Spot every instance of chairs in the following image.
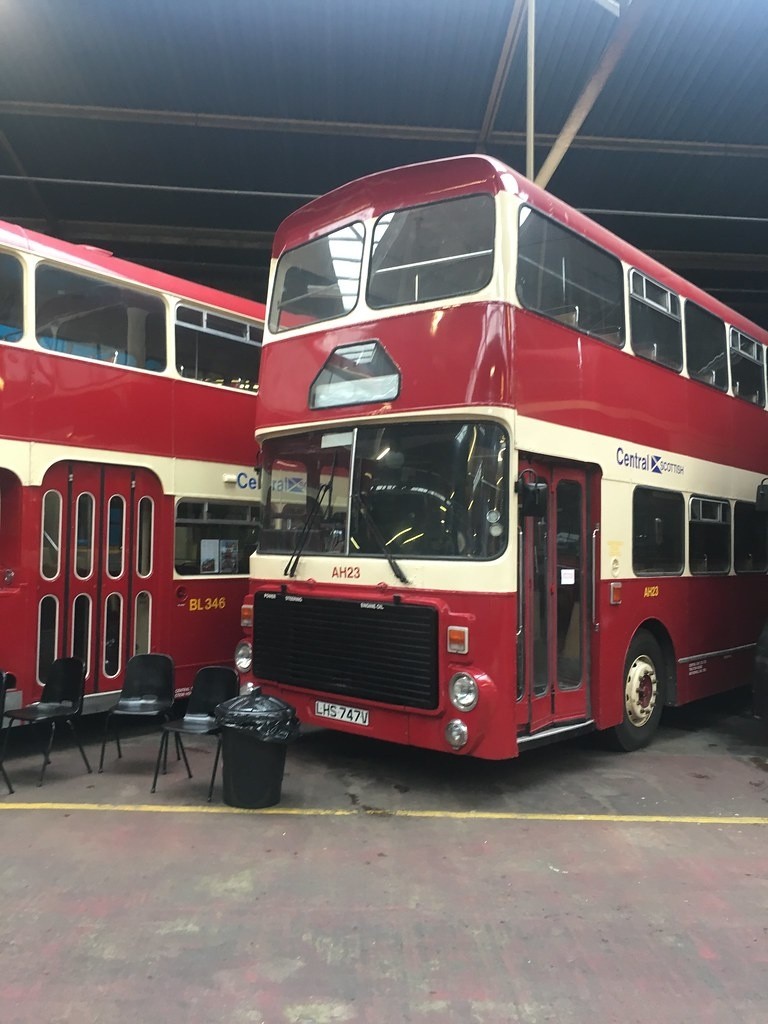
[0,657,93,794]
[151,665,240,801]
[97,653,180,774]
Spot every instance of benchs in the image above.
[540,306,760,405]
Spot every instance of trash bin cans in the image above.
[213,686,299,810]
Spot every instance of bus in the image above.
[0,220,344,730]
[236,152,768,762]
